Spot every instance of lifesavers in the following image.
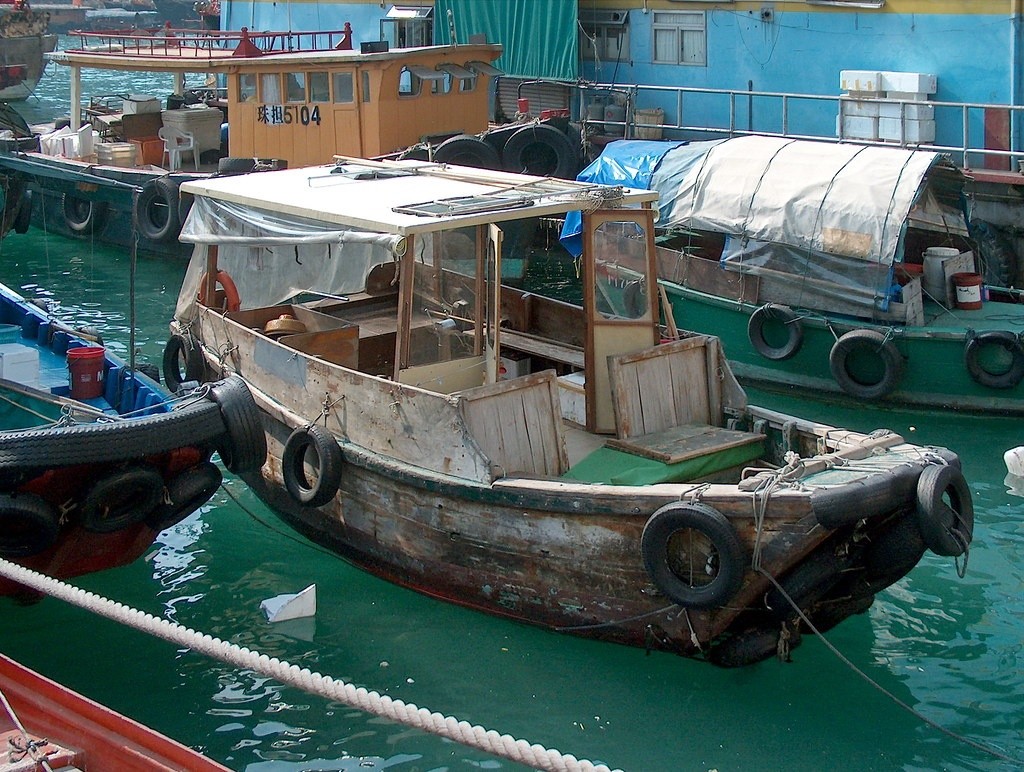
[828,329,903,401]
[16,190,33,234]
[30,299,49,313]
[0,327,268,557]
[937,215,1014,301]
[638,499,748,610]
[964,330,1024,390]
[281,423,344,508]
[746,302,804,361]
[61,191,107,236]
[218,157,288,173]
[431,123,580,181]
[131,178,180,244]
[762,464,975,620]
[200,269,241,313]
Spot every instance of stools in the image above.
[461,326,585,371]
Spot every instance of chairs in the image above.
[168,126,200,169]
[158,126,199,172]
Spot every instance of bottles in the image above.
[981,283,989,302]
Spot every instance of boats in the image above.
[162,151,977,671]
[2,0,1024,422]
[1,185,228,611]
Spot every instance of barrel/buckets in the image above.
[895,263,923,286]
[167,95,185,110]
[0,324,21,344]
[634,109,664,139]
[951,273,982,310]
[922,247,960,303]
[65,347,106,399]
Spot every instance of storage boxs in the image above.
[558,371,588,426]
[836,69,937,143]
[122,98,162,115]
[0,344,38,383]
[499,357,532,380]
[95,135,183,168]
